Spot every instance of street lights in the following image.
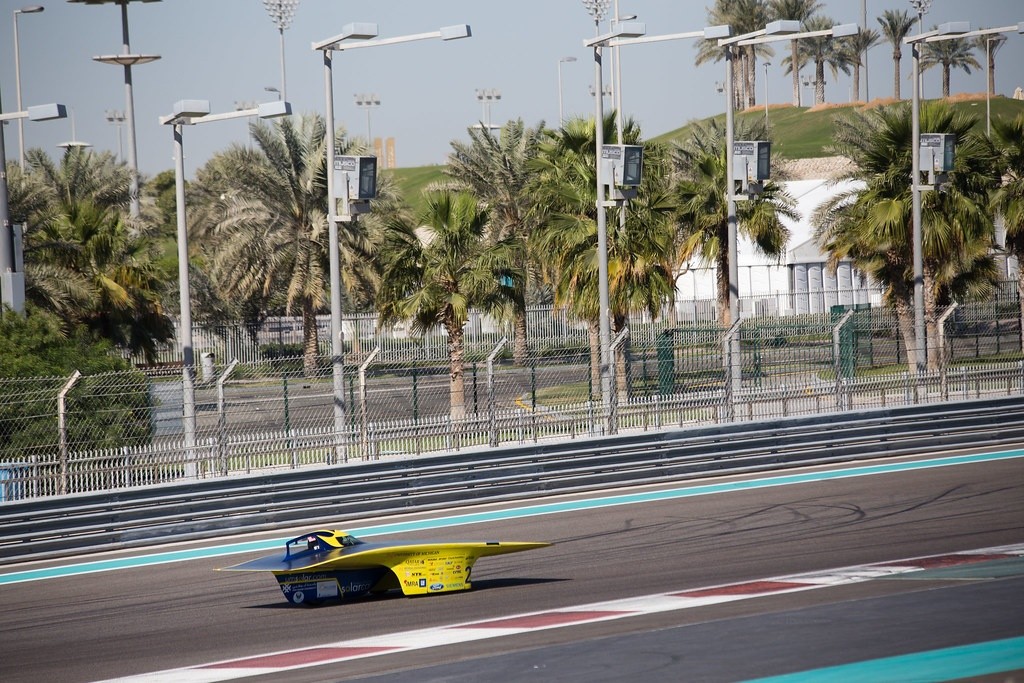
[909,0,932,99]
[264,86,282,101]
[763,62,772,141]
[0,93,67,323]
[902,20,1024,381]
[159,97,293,479]
[313,21,471,464]
[353,92,382,150]
[13,6,45,193]
[987,35,1008,139]
[560,56,578,129]
[106,109,128,164]
[611,14,639,120]
[584,19,733,442]
[716,19,862,419]
[475,87,502,137]
